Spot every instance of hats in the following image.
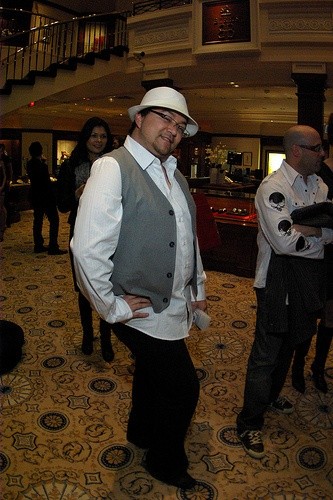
[127,87,199,140]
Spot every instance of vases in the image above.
[209,167,225,185]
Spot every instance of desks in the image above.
[9,180,57,211]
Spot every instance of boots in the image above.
[292,337,312,392]
[78,293,94,355]
[100,317,114,362]
[311,325,333,394]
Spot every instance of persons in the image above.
[70,87,208,489]
[56,117,114,362]
[0,144,13,242]
[292,114,333,394]
[236,126,333,458]
[26,141,67,255]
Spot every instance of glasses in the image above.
[149,109,190,139]
[297,144,328,152]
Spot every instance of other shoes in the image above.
[48,249,67,255]
[143,462,196,489]
[37,246,48,253]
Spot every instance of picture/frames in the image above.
[243,152,253,166]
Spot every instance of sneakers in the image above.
[238,429,265,459]
[268,397,294,414]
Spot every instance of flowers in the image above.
[204,143,228,169]
